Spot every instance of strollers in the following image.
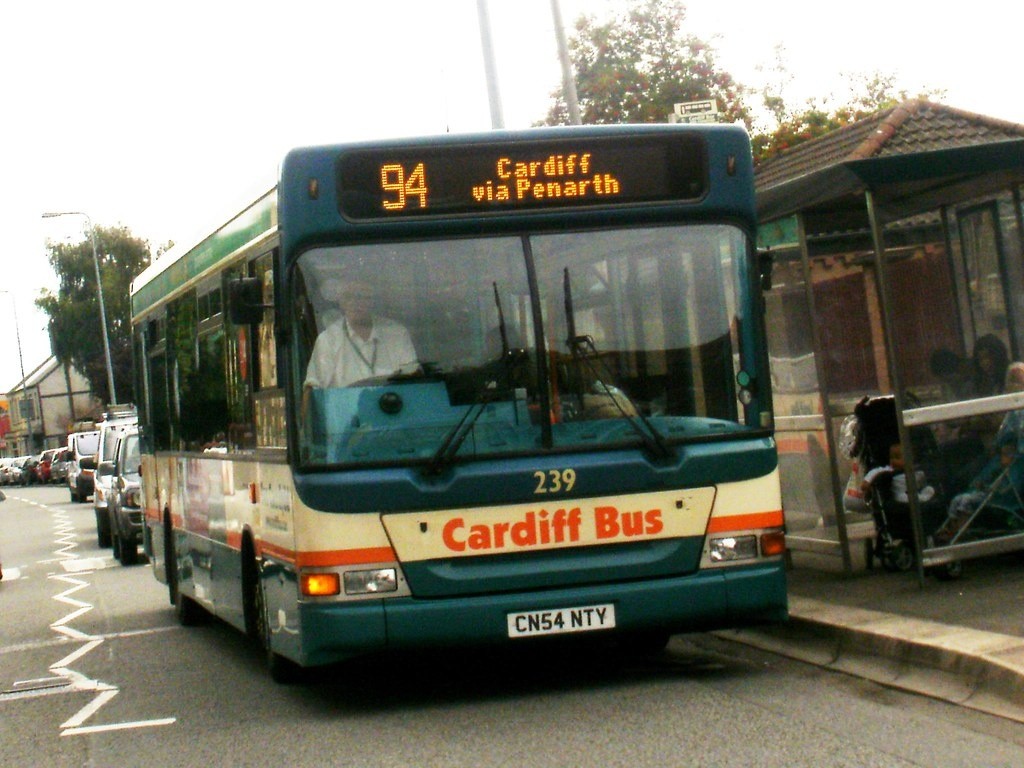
[849,390,949,572]
[924,427,1024,581]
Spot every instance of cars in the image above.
[0,445,71,488]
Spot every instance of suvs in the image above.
[98,431,143,567]
[92,416,140,549]
[65,429,103,504]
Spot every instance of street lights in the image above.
[39,210,117,405]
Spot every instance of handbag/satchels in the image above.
[843,456,867,514]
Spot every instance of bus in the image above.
[127,121,795,689]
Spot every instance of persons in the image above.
[860,334,1018,540]
[301,274,424,425]
